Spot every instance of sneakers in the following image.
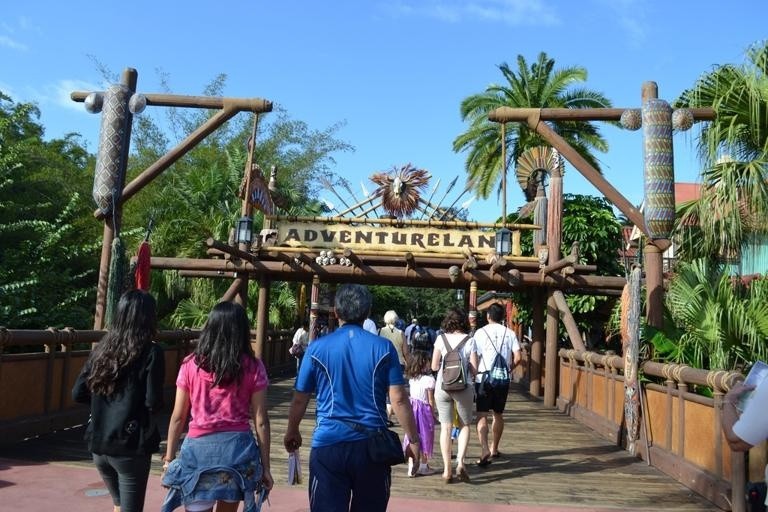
[409,464,436,478]
[475,451,511,469]
[441,468,472,485]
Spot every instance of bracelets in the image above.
[161,454,177,463]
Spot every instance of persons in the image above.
[430,307,479,485]
[402,349,439,478]
[287,301,462,458]
[720,374,767,512]
[71,288,166,512]
[283,282,421,512]
[161,300,275,512]
[472,302,523,467]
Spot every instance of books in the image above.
[732,359,768,413]
[285,443,303,486]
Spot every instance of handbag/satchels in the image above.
[410,326,416,341]
[369,427,405,465]
[291,344,304,358]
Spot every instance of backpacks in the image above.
[414,326,431,351]
[481,327,512,398]
[441,333,472,391]
